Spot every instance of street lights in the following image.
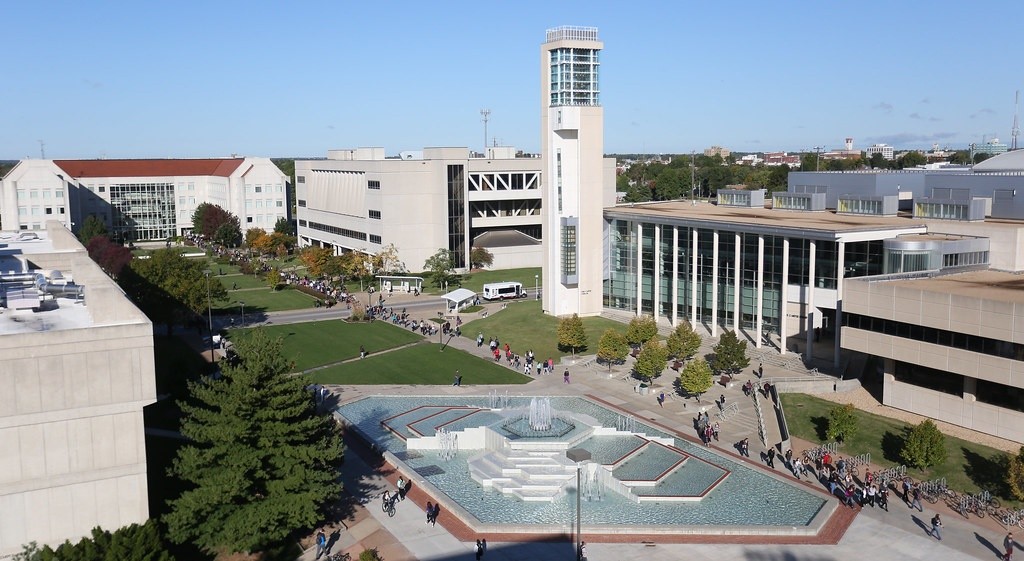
[202,269,216,379]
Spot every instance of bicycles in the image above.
[845,459,860,476]
[382,500,396,517]
[874,466,1024,531]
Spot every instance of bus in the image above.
[481,280,528,300]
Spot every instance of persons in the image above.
[475,294,480,305]
[397,476,405,502]
[190,234,347,301]
[459,284,462,288]
[453,369,462,387]
[382,490,392,510]
[504,343,521,368]
[548,357,554,370]
[1001,532,1014,561]
[476,332,483,349]
[414,288,418,296]
[474,538,486,561]
[442,321,461,337]
[483,312,489,318]
[489,336,499,351]
[563,368,570,384]
[659,363,944,541]
[360,345,365,359]
[543,359,549,375]
[580,541,587,561]
[316,529,329,559]
[426,500,435,528]
[492,346,500,362]
[537,361,542,375]
[524,349,534,375]
[366,293,436,336]
[502,303,506,308]
